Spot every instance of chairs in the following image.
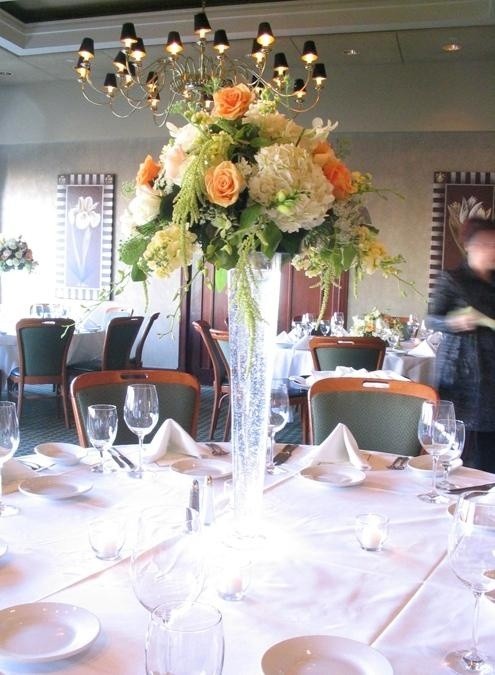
[68,365,200,452]
[191,315,439,456]
[0,302,160,433]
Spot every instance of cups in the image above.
[87,520,128,560]
[146,601,225,675]
[302,311,345,338]
[352,514,391,553]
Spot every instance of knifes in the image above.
[447,483,495,494]
[272,444,298,465]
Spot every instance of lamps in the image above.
[72,0,328,129]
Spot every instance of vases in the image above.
[221,251,284,509]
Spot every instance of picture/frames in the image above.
[427,168,494,322]
[56,172,114,302]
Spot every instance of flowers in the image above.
[0,231,39,272]
[59,73,430,361]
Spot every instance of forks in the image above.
[387,456,406,470]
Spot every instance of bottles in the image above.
[406,313,419,338]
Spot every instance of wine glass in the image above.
[445,491,495,674]
[131,509,207,675]
[268,384,289,466]
[0,400,22,518]
[86,384,159,474]
[386,335,401,353]
[418,399,465,504]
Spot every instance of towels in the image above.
[136,418,214,463]
[308,422,368,469]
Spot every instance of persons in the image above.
[424,218,495,474]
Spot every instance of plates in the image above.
[0,601,101,665]
[173,457,232,481]
[408,455,464,476]
[260,635,395,675]
[19,442,93,501]
[446,503,495,532]
[298,465,368,488]
